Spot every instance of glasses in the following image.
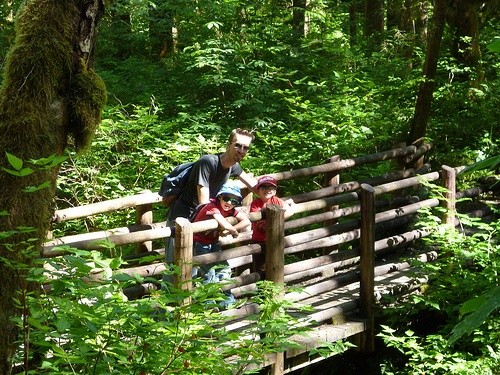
[222,195,239,207]
[258,185,274,191]
[231,141,249,150]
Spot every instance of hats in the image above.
[255,175,277,190]
[216,181,243,199]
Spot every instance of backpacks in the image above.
[158,154,217,208]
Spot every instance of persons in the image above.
[164,128,294,307]
[193,183,251,307]
[249,176,294,281]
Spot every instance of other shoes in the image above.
[209,306,220,315]
[234,297,248,309]
